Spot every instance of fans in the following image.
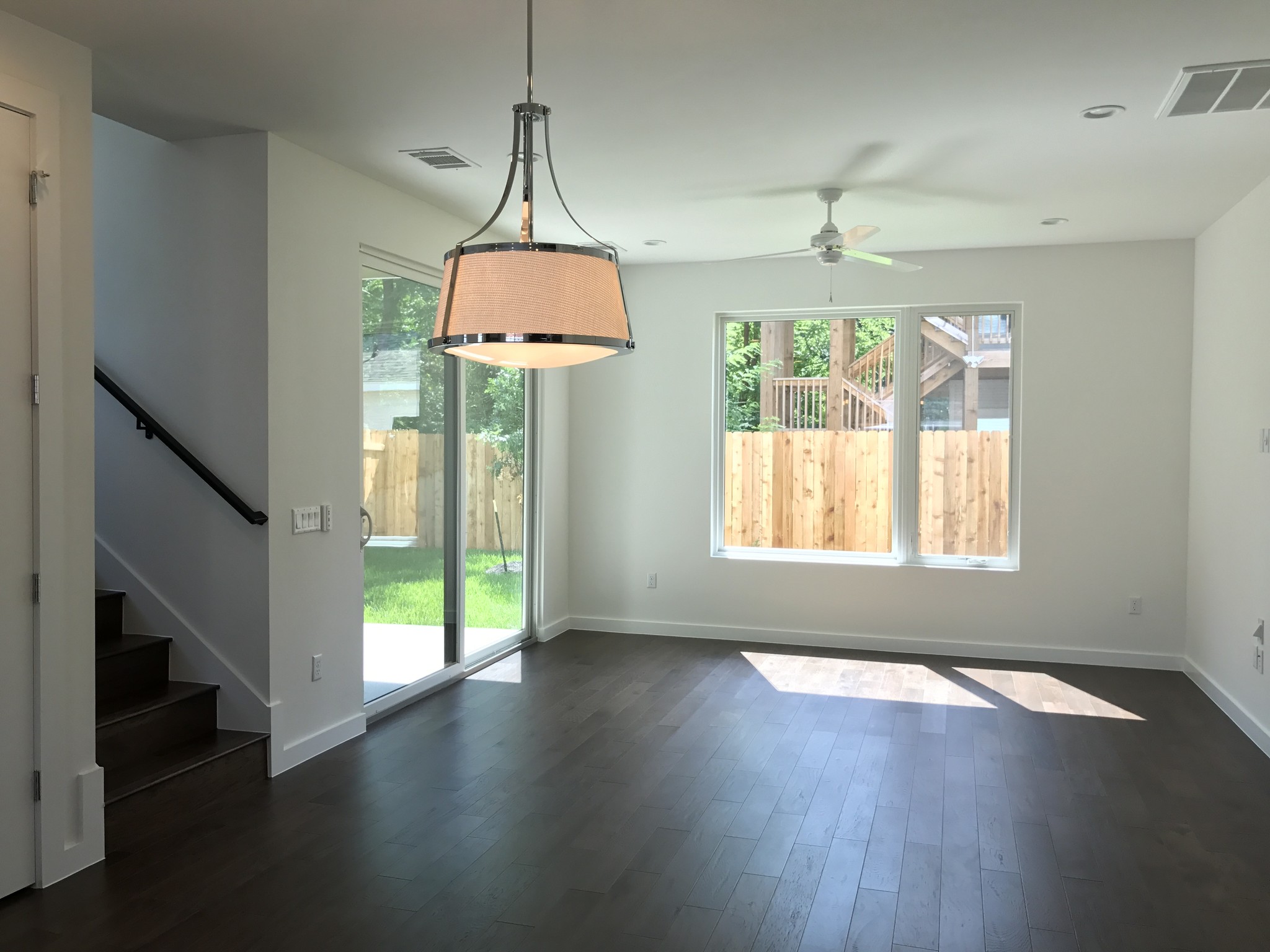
[702,188,922,272]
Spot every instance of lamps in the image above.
[426,0,635,374]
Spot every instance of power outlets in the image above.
[1257,649,1263,673]
[312,654,322,681]
[1128,596,1142,614]
[648,573,656,588]
